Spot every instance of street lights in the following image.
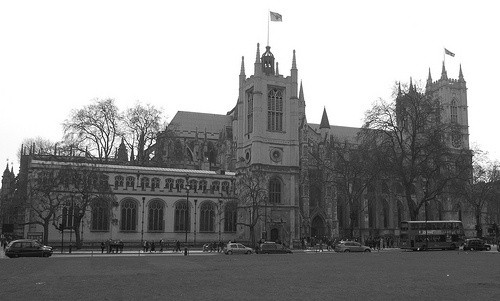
[264,196,269,241]
[185,183,191,242]
[69,188,75,253]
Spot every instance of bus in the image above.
[399,221,465,250]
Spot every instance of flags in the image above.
[270,11,282,22]
[445,48,455,57]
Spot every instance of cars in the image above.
[333,241,371,253]
[223,243,253,255]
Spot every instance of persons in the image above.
[218,242,222,253]
[100,239,124,254]
[143,239,182,253]
[301,233,394,253]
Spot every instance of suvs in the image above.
[463,239,491,251]
[5,239,53,257]
[255,241,292,254]
